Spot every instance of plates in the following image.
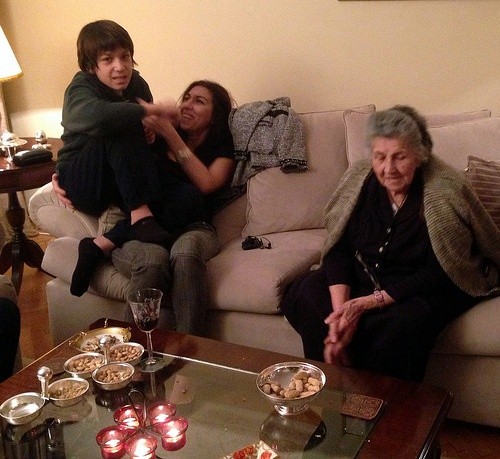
[73,326,131,354]
[222,441,278,459]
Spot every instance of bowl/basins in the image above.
[256,362,326,415]
[109,343,144,366]
[91,361,135,391]
[62,352,105,379]
[0,392,45,425]
[46,377,91,405]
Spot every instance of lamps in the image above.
[0,25,55,236]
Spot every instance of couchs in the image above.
[28,103,500,428]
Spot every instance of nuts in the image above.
[50,346,141,399]
[262,371,322,399]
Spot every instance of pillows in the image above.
[344,110,500,171]
[241,105,376,238]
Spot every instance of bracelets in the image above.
[176,148,192,164]
[374,290,385,308]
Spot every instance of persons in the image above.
[55,21,180,297]
[52,80,236,333]
[283,105,500,385]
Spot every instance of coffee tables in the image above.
[0,317,453,459]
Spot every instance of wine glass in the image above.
[126,288,166,374]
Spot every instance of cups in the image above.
[3,145,16,163]
[95,400,186,459]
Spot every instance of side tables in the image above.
[0,138,64,294]
